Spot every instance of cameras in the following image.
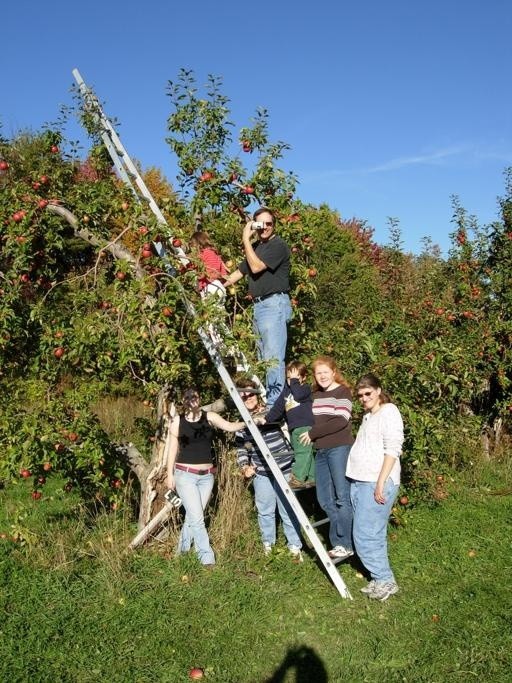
[164,490,183,509]
[251,221,264,230]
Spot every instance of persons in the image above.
[298,354,354,557]
[345,372,405,603]
[253,360,316,489]
[185,231,241,357]
[164,386,251,568]
[236,379,304,564]
[220,207,294,421]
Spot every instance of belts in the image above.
[253,291,286,302]
[175,464,218,475]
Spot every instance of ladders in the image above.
[72,66,357,601]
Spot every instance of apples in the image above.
[189,668,204,679]
[0,140,512,583]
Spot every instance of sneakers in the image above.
[288,475,316,489]
[287,544,304,563]
[328,543,355,558]
[359,575,399,603]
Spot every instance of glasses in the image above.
[357,389,376,398]
